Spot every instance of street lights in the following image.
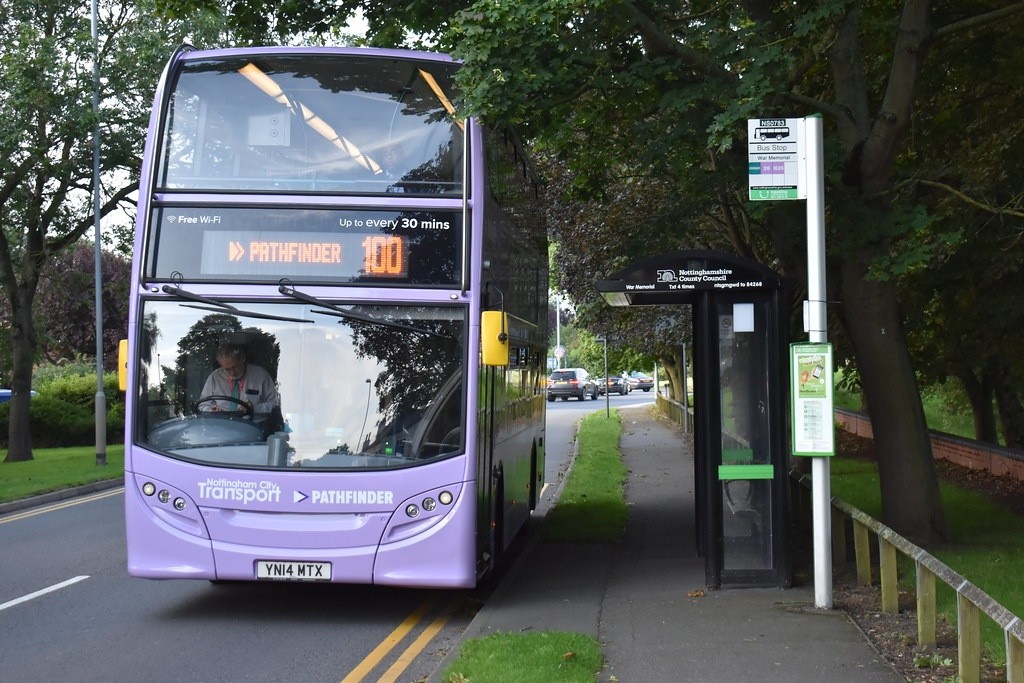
[595,339,609,417]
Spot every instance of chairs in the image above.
[246,340,289,428]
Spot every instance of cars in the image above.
[597,370,654,396]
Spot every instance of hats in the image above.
[218,333,245,345]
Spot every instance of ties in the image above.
[230,378,239,411]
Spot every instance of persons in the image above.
[196,336,280,429]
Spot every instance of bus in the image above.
[118,43,549,589]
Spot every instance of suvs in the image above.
[547,368,598,402]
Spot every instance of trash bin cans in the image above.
[0,389,36,402]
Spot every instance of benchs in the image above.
[724,479,764,531]
